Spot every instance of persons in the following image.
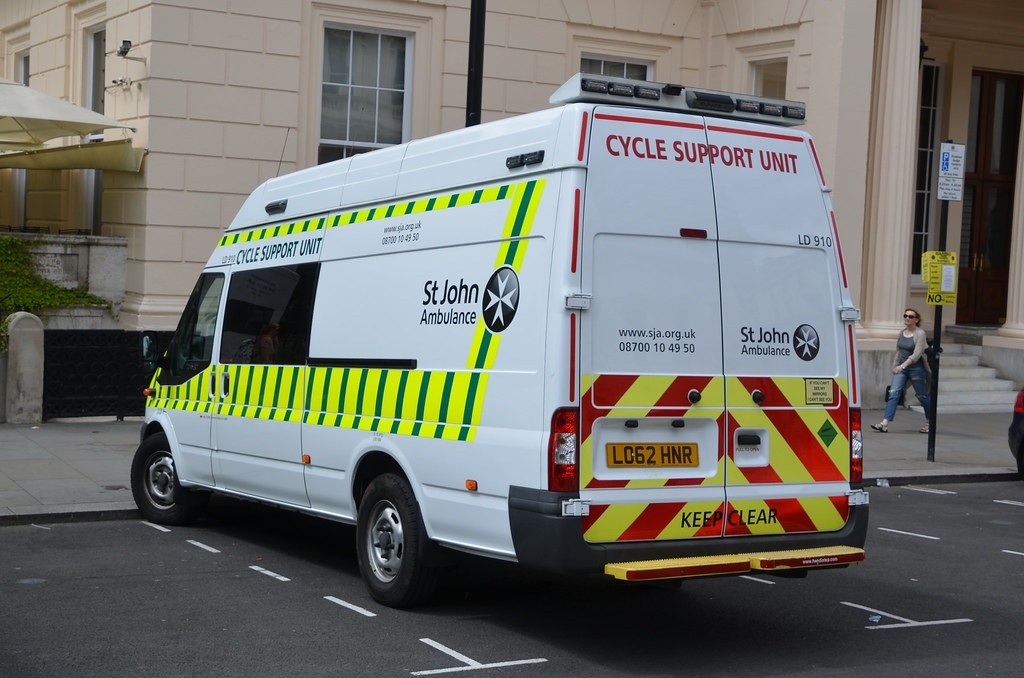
[233,323,281,364]
[870,309,933,434]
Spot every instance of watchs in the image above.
[900,365,905,371]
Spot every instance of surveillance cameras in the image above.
[116,40,132,56]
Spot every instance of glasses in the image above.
[903,314,917,319]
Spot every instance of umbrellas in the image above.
[0,76,137,153]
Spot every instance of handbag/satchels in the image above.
[885,385,903,406]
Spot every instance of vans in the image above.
[130,69,867,613]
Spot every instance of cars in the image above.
[1006,389,1024,479]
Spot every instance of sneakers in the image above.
[920,422,938,433]
[870,422,888,433]
